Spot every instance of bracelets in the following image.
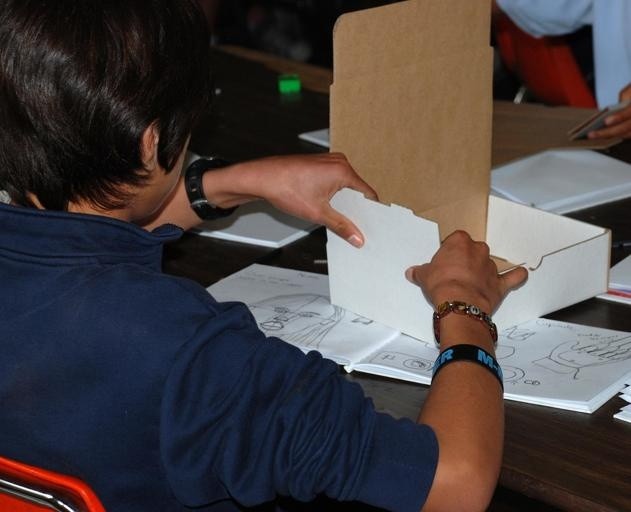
[185,155,239,220]
[434,300,497,342]
[431,344,503,389]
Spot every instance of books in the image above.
[205,261,631,417]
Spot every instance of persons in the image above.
[0,1,528,512]
[491,0,631,141]
